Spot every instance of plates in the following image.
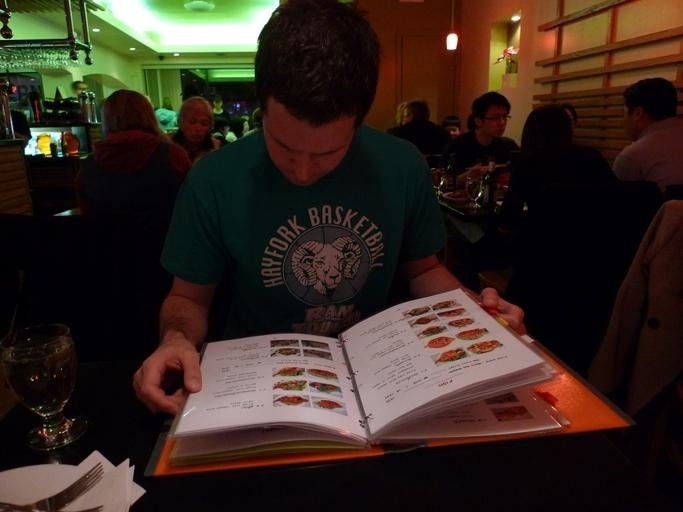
[441,192,466,205]
[0,464,80,512]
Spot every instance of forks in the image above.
[0,461,105,512]
[0,501,104,512]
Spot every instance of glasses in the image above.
[487,115,511,120]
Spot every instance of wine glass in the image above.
[463,168,484,214]
[1,48,80,73]
[0,320,89,450]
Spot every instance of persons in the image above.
[2,89,232,346]
[128,2,530,412]
[387,90,520,174]
[502,78,681,373]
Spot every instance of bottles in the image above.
[431,168,438,193]
[484,161,498,217]
[77,91,98,124]
[28,91,42,122]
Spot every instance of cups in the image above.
[440,171,457,192]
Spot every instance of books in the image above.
[166,283,572,469]
